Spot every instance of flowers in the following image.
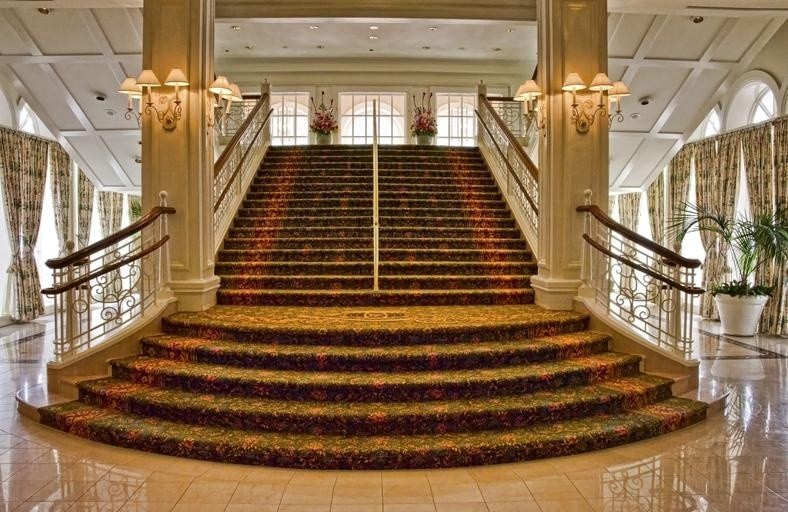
[410,92,438,137]
[309,91,338,133]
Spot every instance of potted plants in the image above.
[417,135,433,145]
[661,198,788,336]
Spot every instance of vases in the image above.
[709,337,766,383]
[315,132,331,145]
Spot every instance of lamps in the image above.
[208,75,243,131]
[135,69,190,132]
[512,80,544,131]
[116,78,142,128]
[560,73,614,134]
[608,81,631,130]
